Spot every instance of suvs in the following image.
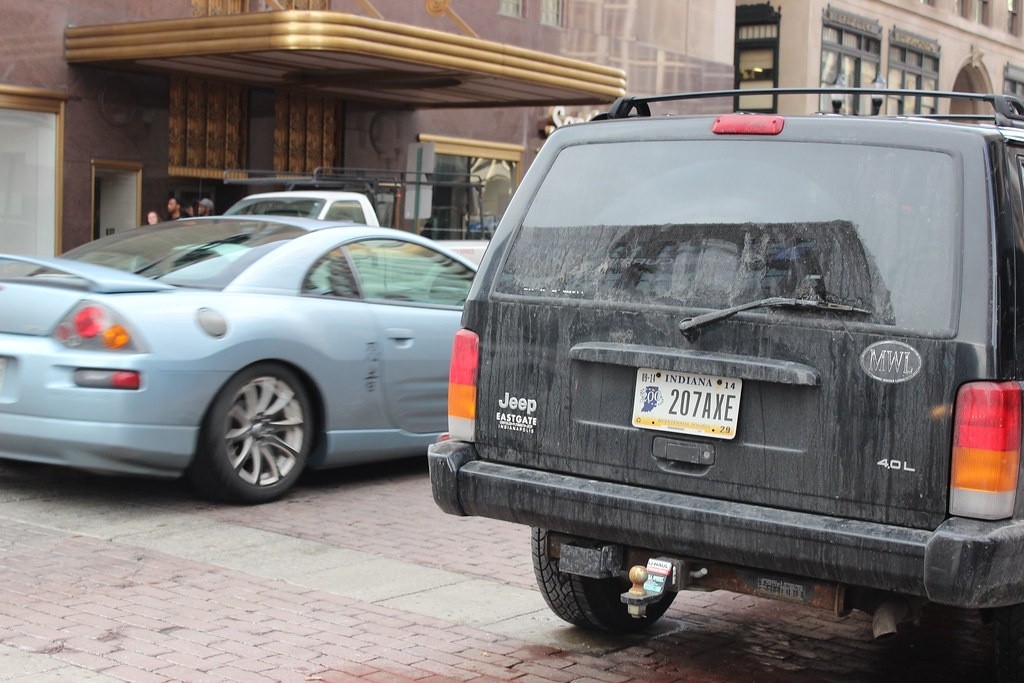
[425,87,1024,654]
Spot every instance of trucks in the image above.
[221,192,491,274]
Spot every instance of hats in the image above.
[198,198,213,209]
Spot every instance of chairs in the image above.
[327,256,360,298]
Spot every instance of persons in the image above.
[165,197,215,222]
[145,208,162,225]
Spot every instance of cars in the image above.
[2,214,479,506]
